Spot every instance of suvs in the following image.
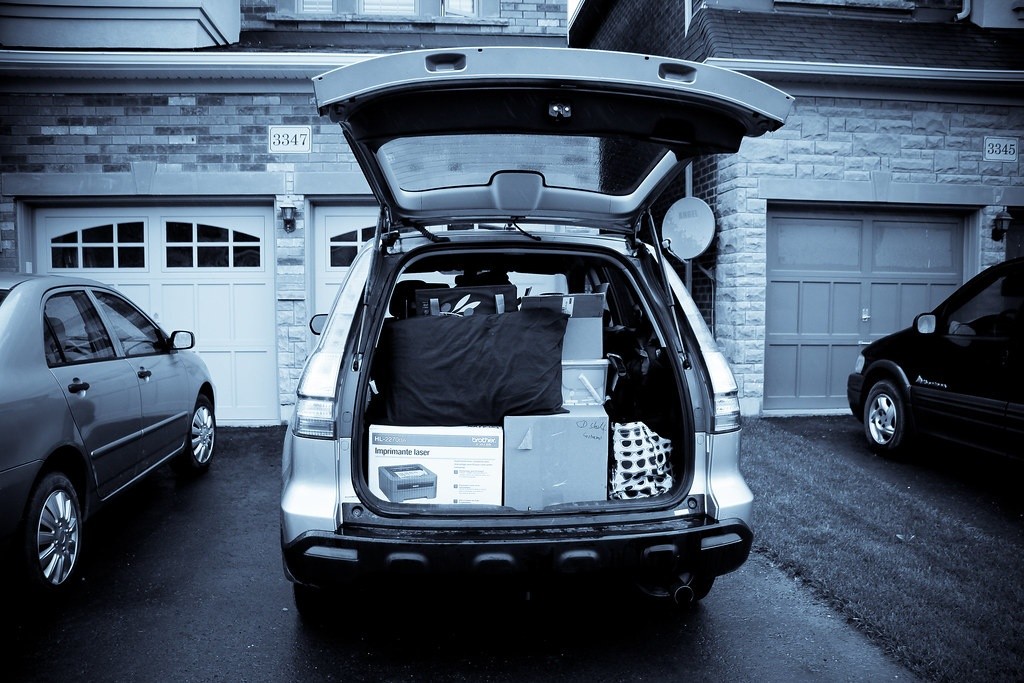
[277,46,795,629]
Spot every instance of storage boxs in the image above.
[562,360,609,401]
[521,292,604,317]
[503,404,609,505]
[368,424,504,507]
[563,317,603,361]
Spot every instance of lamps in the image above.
[993,205,1013,240]
[280,196,298,234]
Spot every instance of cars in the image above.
[0,274,217,616]
[847,255,1024,478]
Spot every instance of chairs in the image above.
[388,278,427,321]
[43,316,84,362]
